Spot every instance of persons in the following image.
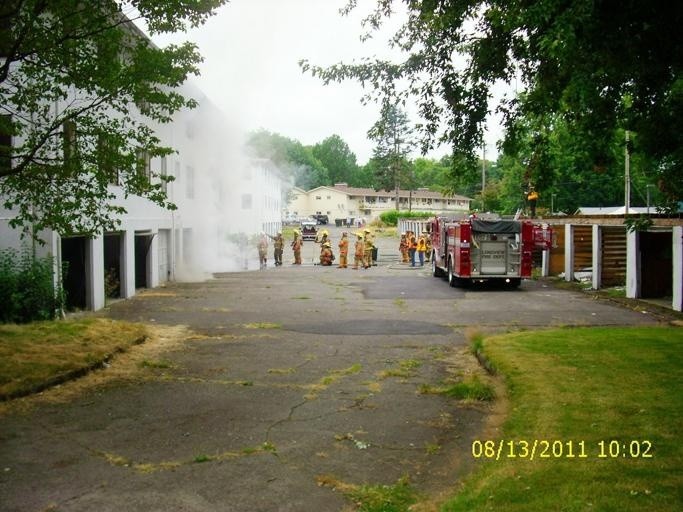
[522,181,538,217]
[263,231,284,266]
[314,231,330,254]
[254,231,268,268]
[336,232,349,269]
[345,217,351,228]
[289,229,303,264]
[397,229,432,268]
[318,242,334,265]
[351,228,378,270]
[356,216,362,228]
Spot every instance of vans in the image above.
[307,215,329,225]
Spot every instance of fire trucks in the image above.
[429,214,554,290]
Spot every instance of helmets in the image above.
[275,228,431,237]
